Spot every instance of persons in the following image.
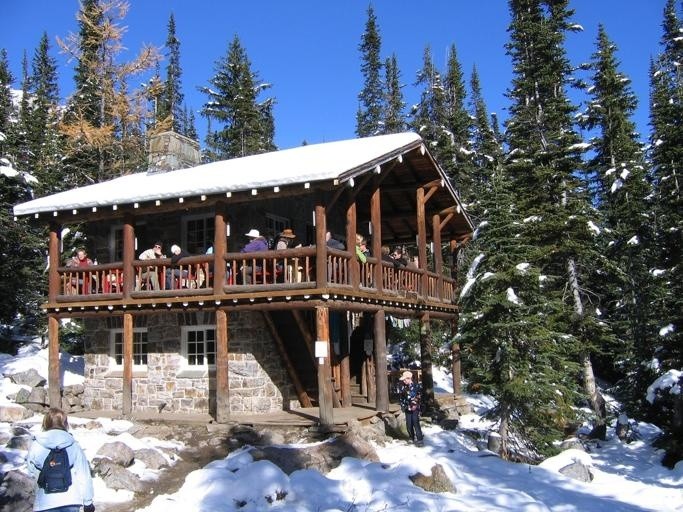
[398,370,424,446]
[64,246,100,295]
[135,227,414,290]
[24,408,95,511]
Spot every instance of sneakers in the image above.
[403,439,424,449]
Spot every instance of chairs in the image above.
[61,259,370,296]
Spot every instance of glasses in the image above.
[154,245,161,249]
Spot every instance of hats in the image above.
[397,371,413,381]
[278,229,296,240]
[242,228,262,239]
